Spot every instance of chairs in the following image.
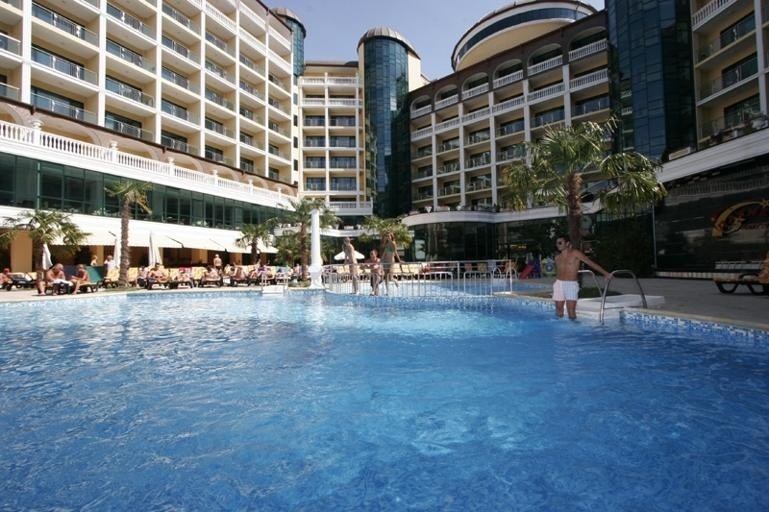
[465,262,518,279]
[3,264,308,301]
[333,259,426,282]
[714,260,769,295]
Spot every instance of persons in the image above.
[104,255,116,268]
[135,262,302,293]
[34,260,91,295]
[0,267,15,291]
[212,253,222,266]
[552,235,616,320]
[90,253,100,266]
[342,231,403,296]
